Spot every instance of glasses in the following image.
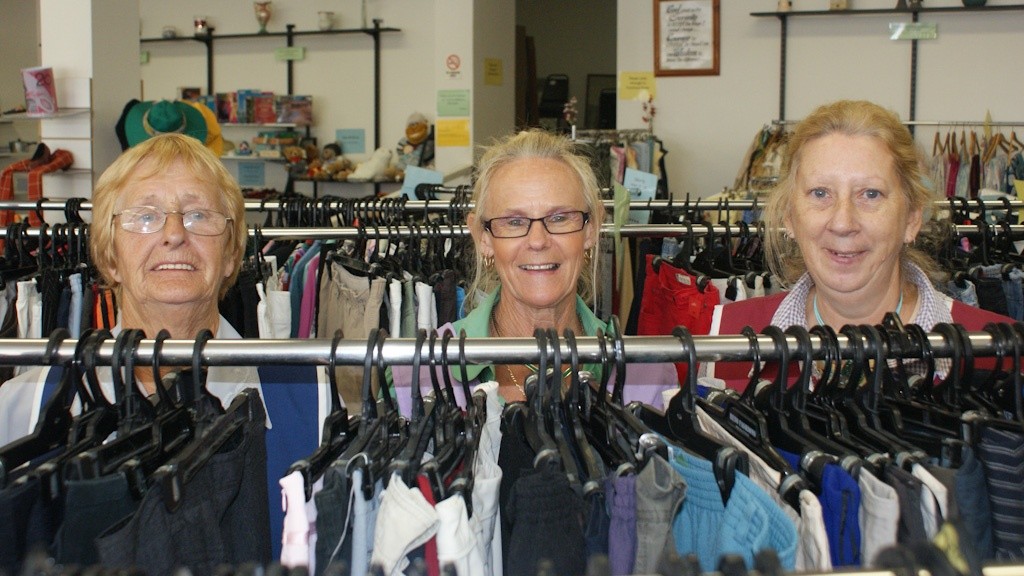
[112,205,234,236]
[484,210,590,237]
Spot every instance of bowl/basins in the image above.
[962,0,986,6]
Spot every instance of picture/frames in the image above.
[653,0,720,75]
[584,74,616,128]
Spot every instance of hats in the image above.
[124,101,207,146]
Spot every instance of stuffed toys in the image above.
[289,112,434,182]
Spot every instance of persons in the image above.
[0,133,277,448]
[693,99,1024,398]
[370,130,685,422]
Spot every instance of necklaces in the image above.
[504,358,528,399]
[813,290,909,330]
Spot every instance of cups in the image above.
[830,0,847,10]
[777,0,791,12]
[319,12,333,29]
[195,16,207,35]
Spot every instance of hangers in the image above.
[0,120,1024,514]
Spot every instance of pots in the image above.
[9,138,37,153]
